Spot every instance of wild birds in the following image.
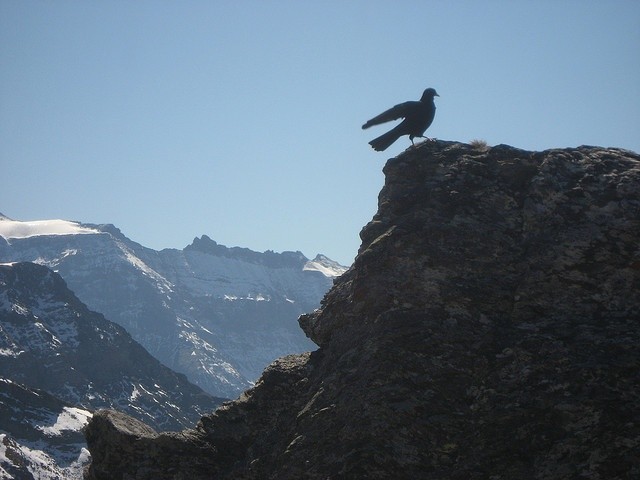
[362,88,440,152]
